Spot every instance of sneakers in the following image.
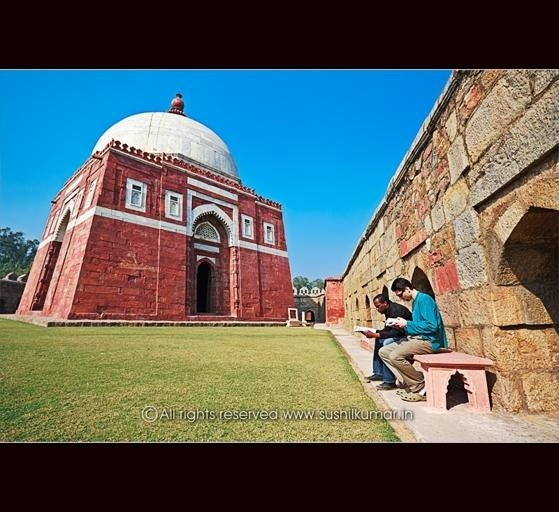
[376,382,395,389]
[367,375,383,381]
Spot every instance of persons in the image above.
[376,277,448,402]
[360,293,413,391]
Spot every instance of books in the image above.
[354,325,376,334]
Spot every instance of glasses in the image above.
[397,290,403,297]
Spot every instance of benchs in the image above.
[408,348,493,410]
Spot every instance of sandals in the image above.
[396,387,410,395]
[401,392,426,402]
